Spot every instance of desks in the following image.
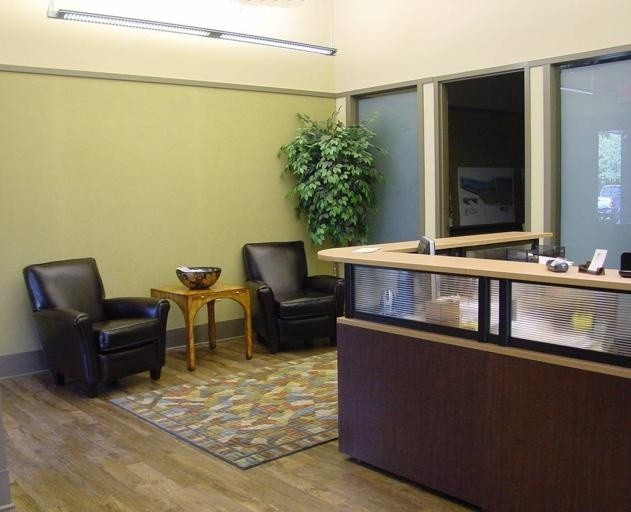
[151,284,255,372]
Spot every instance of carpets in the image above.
[107,350,340,471]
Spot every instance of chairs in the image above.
[244,240,348,354]
[22,258,169,397]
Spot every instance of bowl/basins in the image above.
[176,266,222,290]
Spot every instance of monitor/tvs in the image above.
[451,159,522,234]
[415,236,437,301]
